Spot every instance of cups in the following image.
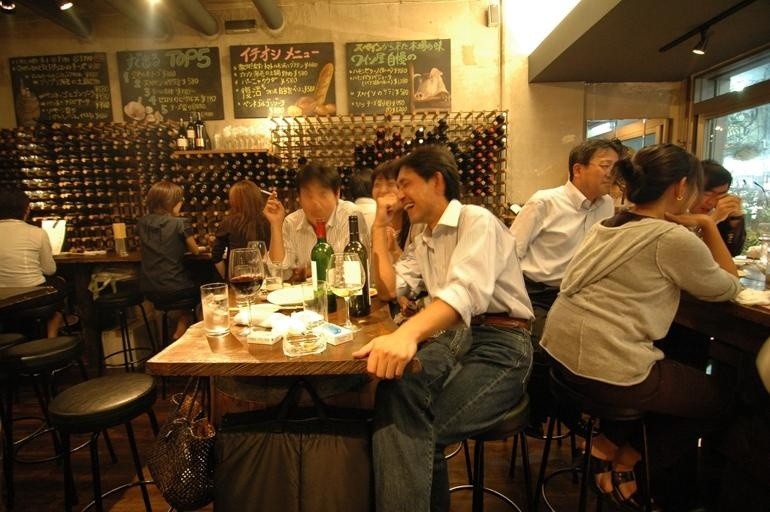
[264,260,284,292]
[300,277,329,325]
[200,282,230,340]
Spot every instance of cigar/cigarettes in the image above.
[260,189,278,198]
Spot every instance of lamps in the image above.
[693,28,713,56]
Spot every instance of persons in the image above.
[372,159,410,253]
[539,142,744,510]
[213,180,272,284]
[507,140,620,334]
[137,180,220,337]
[351,144,535,511]
[683,159,743,269]
[0,185,66,342]
[345,169,375,228]
[263,162,369,279]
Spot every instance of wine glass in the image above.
[756,221,770,266]
[326,251,367,332]
[228,240,267,337]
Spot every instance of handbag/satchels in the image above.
[147,375,219,512]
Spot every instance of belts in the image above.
[471,316,530,330]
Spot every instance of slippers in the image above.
[576,448,619,506]
[612,471,652,511]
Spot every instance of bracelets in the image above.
[729,215,743,221]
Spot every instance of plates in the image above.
[268,288,314,306]
[123,105,146,121]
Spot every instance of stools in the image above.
[538,396,654,512]
[508,354,579,486]
[155,300,201,355]
[446,391,535,511]
[95,292,157,375]
[49,374,176,512]
[9,336,117,476]
[0,333,55,457]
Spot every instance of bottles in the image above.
[311,219,337,314]
[0,113,509,255]
[16,93,41,121]
[342,217,371,317]
